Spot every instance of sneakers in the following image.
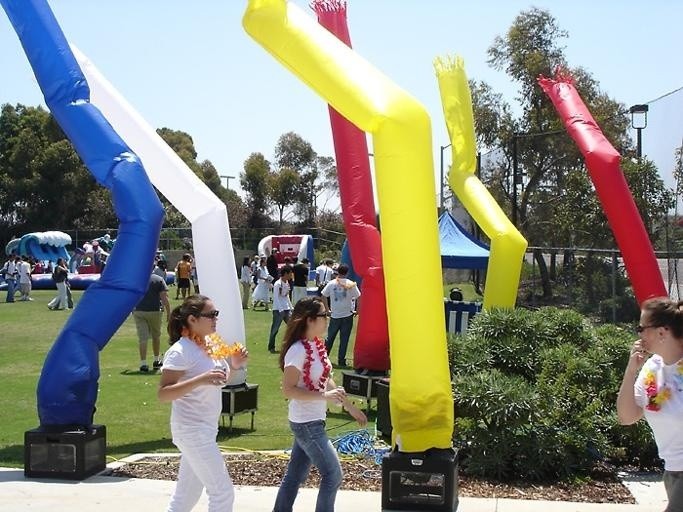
[153,360,162,369]
[140,365,149,373]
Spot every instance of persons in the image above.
[269,294,368,511]
[1,254,75,311]
[615,295,683,511]
[239,248,361,367]
[132,262,170,373]
[153,253,200,299]
[156,291,247,510]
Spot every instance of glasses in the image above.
[635,324,659,333]
[317,312,327,318]
[200,311,219,318]
